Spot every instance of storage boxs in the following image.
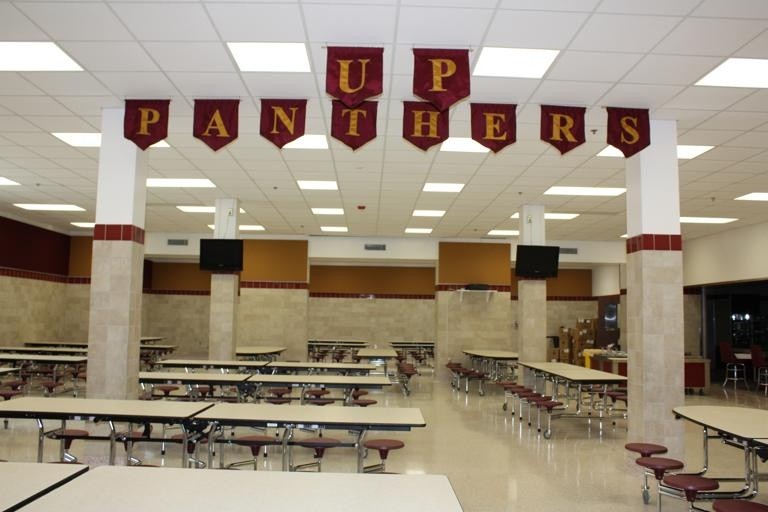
[550,318,622,367]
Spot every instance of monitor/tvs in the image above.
[515,244,560,278]
[200,238,243,272]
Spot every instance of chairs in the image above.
[750,344,767,394]
[717,341,750,392]
